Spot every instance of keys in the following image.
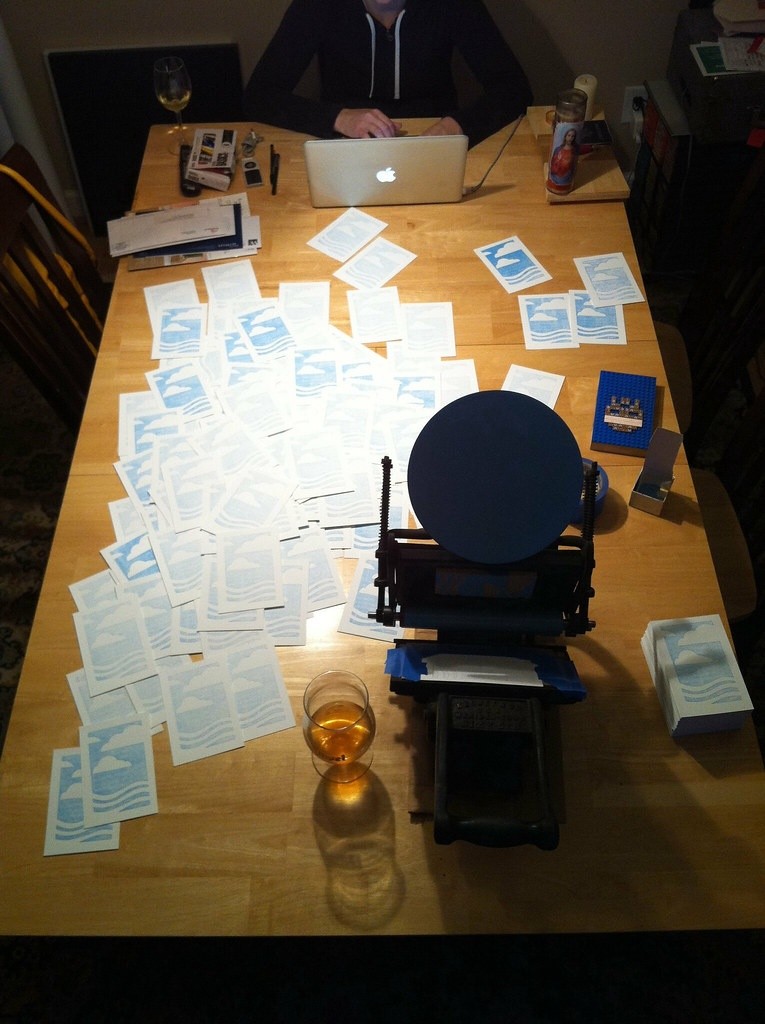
[240,130,265,188]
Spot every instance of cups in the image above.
[546,88,589,195]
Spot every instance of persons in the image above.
[242,0,535,138]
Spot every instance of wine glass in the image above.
[153,56,192,157]
[301,669,376,783]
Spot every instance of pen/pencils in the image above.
[269,144,280,196]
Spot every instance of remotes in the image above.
[180,144,201,197]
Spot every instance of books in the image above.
[589,370,658,458]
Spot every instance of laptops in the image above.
[303,135,469,209]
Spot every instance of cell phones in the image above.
[243,158,264,188]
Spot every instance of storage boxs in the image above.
[640,614,755,736]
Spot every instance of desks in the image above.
[634,7,765,270]
[0,106,765,938]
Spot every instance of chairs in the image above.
[653,145,765,678]
[1,141,110,430]
